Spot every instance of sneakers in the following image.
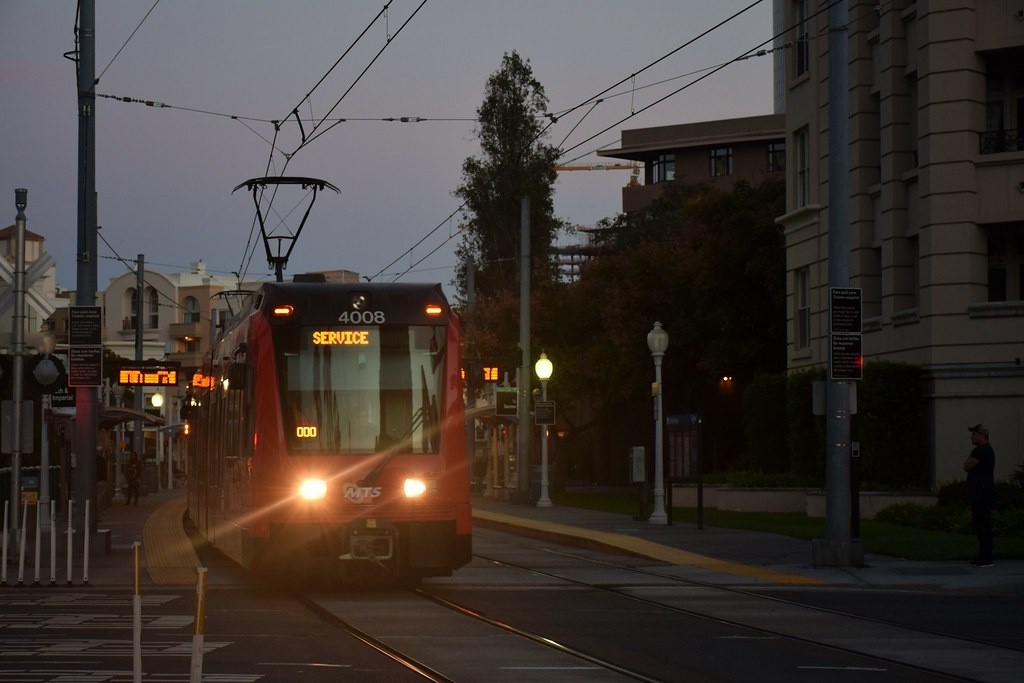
[969,557,994,568]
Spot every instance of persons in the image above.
[125,450,142,507]
[964,422,996,567]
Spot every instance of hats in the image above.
[967,424,990,436]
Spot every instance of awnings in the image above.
[99,407,165,431]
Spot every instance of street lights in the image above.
[535,353,554,508]
[646,322,669,525]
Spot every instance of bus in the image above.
[180,282,473,583]
